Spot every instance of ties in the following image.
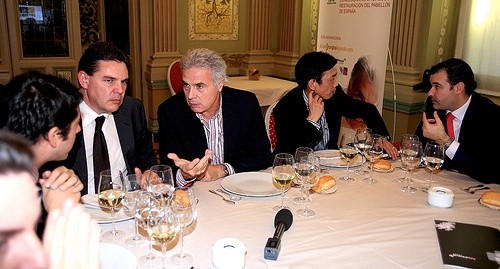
[445,113,456,140]
[93,115,114,194]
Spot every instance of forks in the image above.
[458,184,490,194]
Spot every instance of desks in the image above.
[223,76,298,120]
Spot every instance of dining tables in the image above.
[101,156,500,269]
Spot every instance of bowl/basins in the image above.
[427,186,454,208]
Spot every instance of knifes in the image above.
[209,189,235,205]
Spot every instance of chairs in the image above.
[264,100,277,153]
[166,60,183,96]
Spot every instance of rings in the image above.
[392,147,395,149]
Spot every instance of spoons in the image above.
[217,189,241,200]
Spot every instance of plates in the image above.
[220,171,289,197]
[308,150,366,168]
[80,193,135,225]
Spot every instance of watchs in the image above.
[441,138,453,152]
[222,164,229,177]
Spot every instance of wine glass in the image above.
[272,147,321,217]
[338,128,383,184]
[397,134,444,194]
[98,165,198,269]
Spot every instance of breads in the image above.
[481,191,500,206]
[374,159,391,170]
[311,175,336,193]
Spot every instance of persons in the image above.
[414,58,500,185]
[0,40,168,269]
[338,56,378,149]
[157,47,272,191]
[272,51,401,165]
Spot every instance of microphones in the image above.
[264,209,293,260]
